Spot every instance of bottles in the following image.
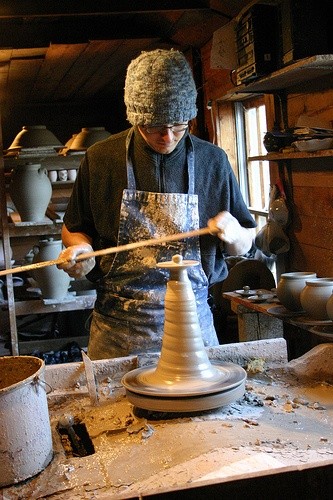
[276,271,318,313]
[300,277,333,320]
[31,237,70,298]
[7,161,52,222]
[325,293,333,320]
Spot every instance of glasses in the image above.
[141,120,192,135]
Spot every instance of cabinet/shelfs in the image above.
[0,148,99,361]
[227,53,333,161]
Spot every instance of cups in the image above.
[48,169,77,182]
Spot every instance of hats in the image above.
[123,48,198,125]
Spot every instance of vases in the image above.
[300,277,333,320]
[276,272,318,313]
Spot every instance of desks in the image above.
[222,288,333,343]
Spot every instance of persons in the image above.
[56,48,258,360]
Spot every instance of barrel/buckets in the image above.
[0,355,54,489]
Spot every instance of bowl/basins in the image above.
[3,125,65,158]
[291,137,333,151]
[65,127,112,154]
